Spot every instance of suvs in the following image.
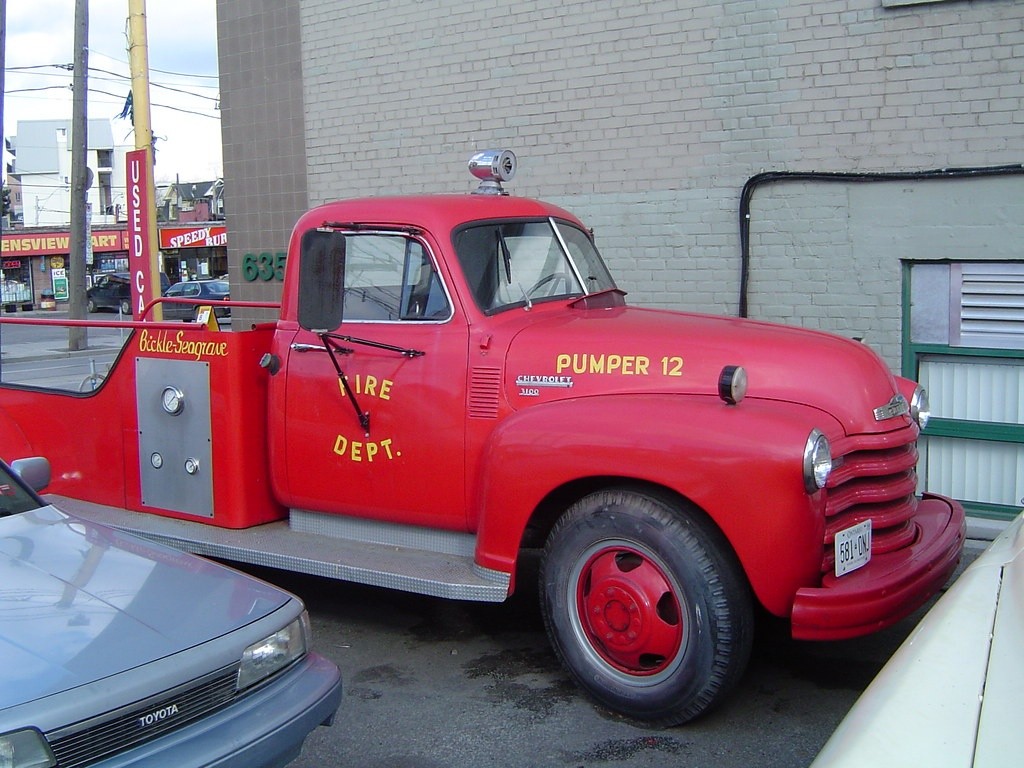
[85,272,172,315]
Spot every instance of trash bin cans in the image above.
[41,293,57,310]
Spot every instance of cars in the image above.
[0,452,345,768]
[162,280,229,322]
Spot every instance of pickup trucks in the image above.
[0,151,970,727]
